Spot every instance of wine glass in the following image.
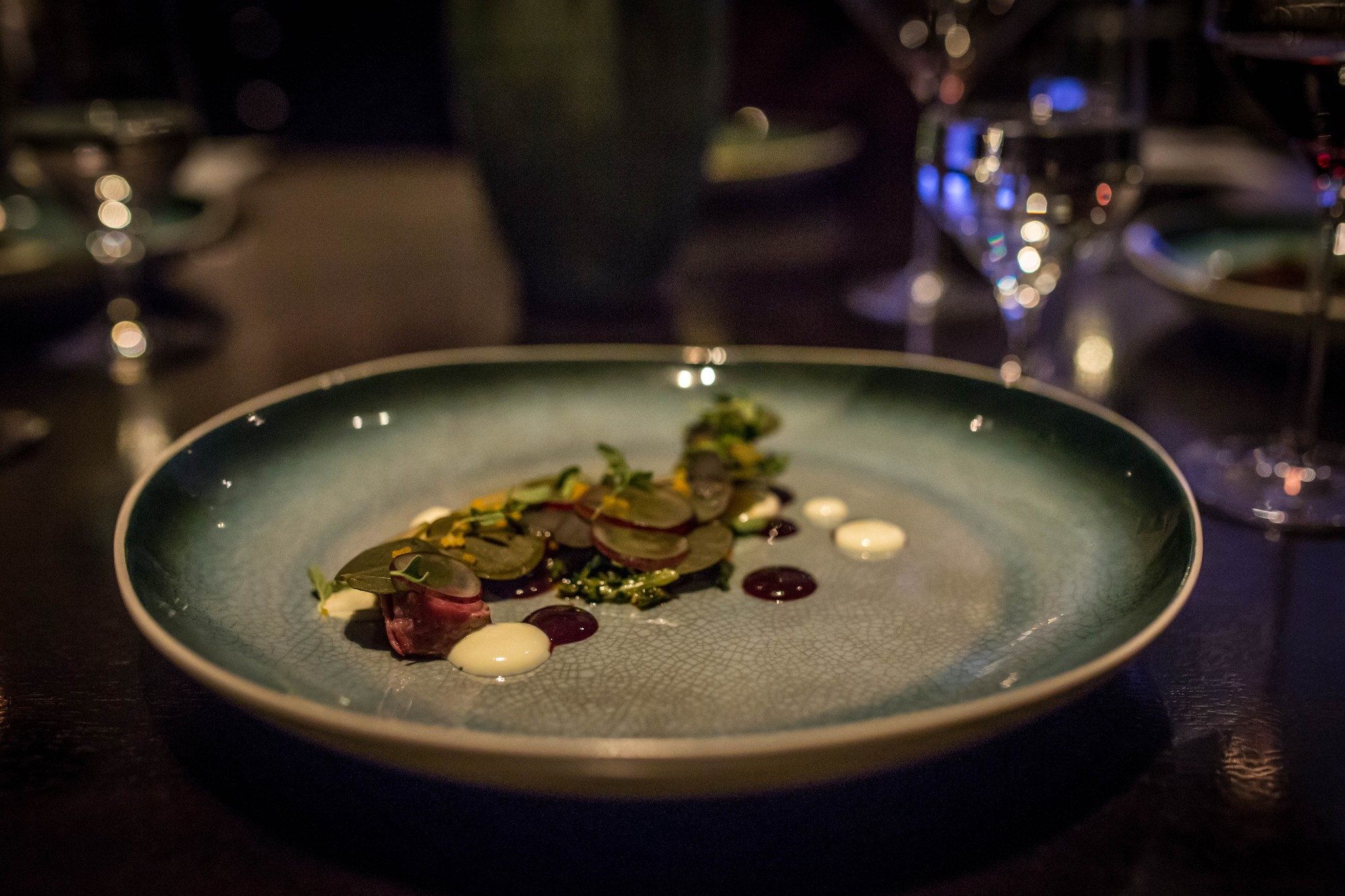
[913,13,1150,385]
[12,15,199,372]
[1178,0,1345,530]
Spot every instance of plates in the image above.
[1126,210,1345,325]
[0,164,244,297]
[114,344,1203,801]
[708,125,855,188]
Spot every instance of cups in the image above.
[453,0,725,322]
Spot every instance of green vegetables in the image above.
[305,390,789,605]
